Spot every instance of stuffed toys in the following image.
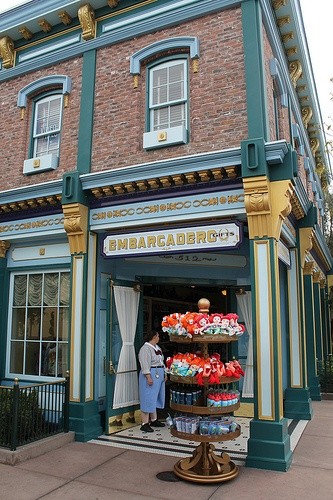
[161,308,246,382]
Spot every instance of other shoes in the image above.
[140,422,153,432]
[150,419,165,427]
[126,416,135,423]
[109,419,122,426]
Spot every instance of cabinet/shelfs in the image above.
[159,298,246,486]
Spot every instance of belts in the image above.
[150,365,164,370]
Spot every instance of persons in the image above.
[137,332,166,432]
